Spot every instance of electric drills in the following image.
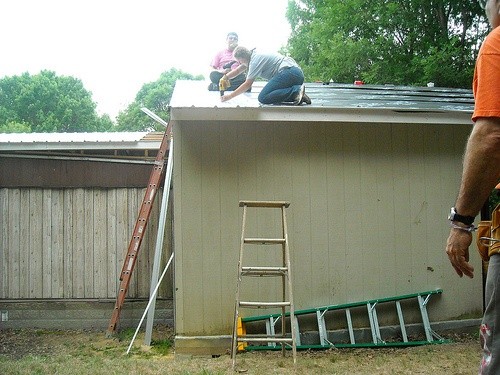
[219,80,231,100]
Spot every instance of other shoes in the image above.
[208,83,218,91]
[295,84,311,105]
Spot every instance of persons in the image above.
[208,32,251,92]
[219,46,311,106]
[445,0,500,375]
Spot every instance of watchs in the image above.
[448,207,476,227]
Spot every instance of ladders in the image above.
[232,201,454,367]
[106,117,175,336]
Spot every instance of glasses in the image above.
[476,0,496,9]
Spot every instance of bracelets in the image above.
[223,75,229,81]
[449,221,475,232]
